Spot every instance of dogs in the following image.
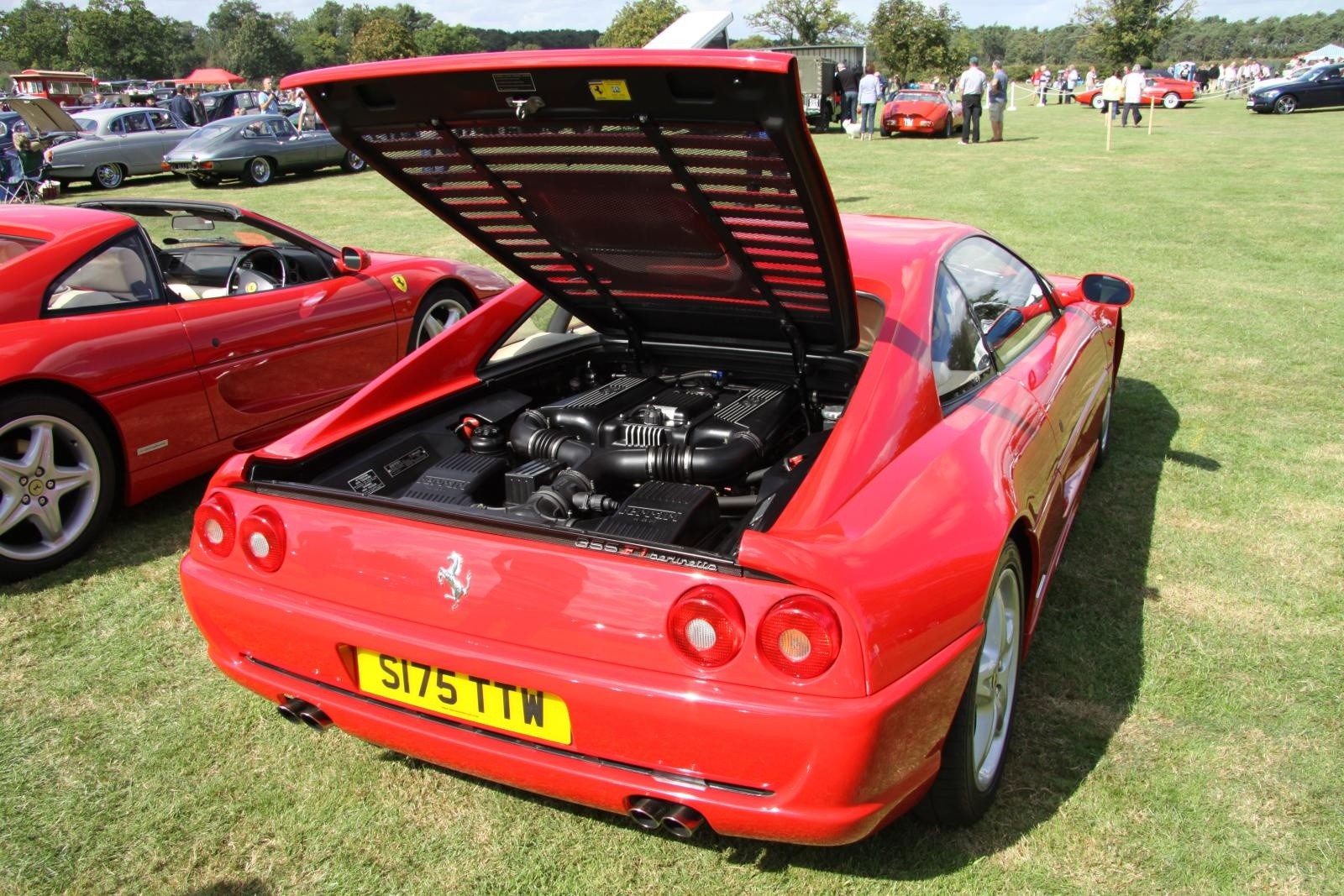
[842,119,861,139]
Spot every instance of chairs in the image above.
[0,151,50,203]
[67,246,154,304]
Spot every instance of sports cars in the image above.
[880,87,964,138]
[174,47,1137,848]
[1,197,518,586]
[1069,68,1204,110]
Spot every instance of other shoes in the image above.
[987,137,1003,142]
[958,141,968,144]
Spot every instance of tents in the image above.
[175,69,246,89]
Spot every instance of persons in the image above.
[735,131,791,208]
[988,59,1008,142]
[832,54,858,134]
[958,56,987,146]
[889,69,957,98]
[258,76,317,131]
[1101,69,1122,128]
[859,64,888,140]
[1028,55,1344,109]
[9,131,42,203]
[1121,63,1146,128]
[58,84,209,128]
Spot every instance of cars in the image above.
[0,85,373,191]
[1244,60,1344,115]
